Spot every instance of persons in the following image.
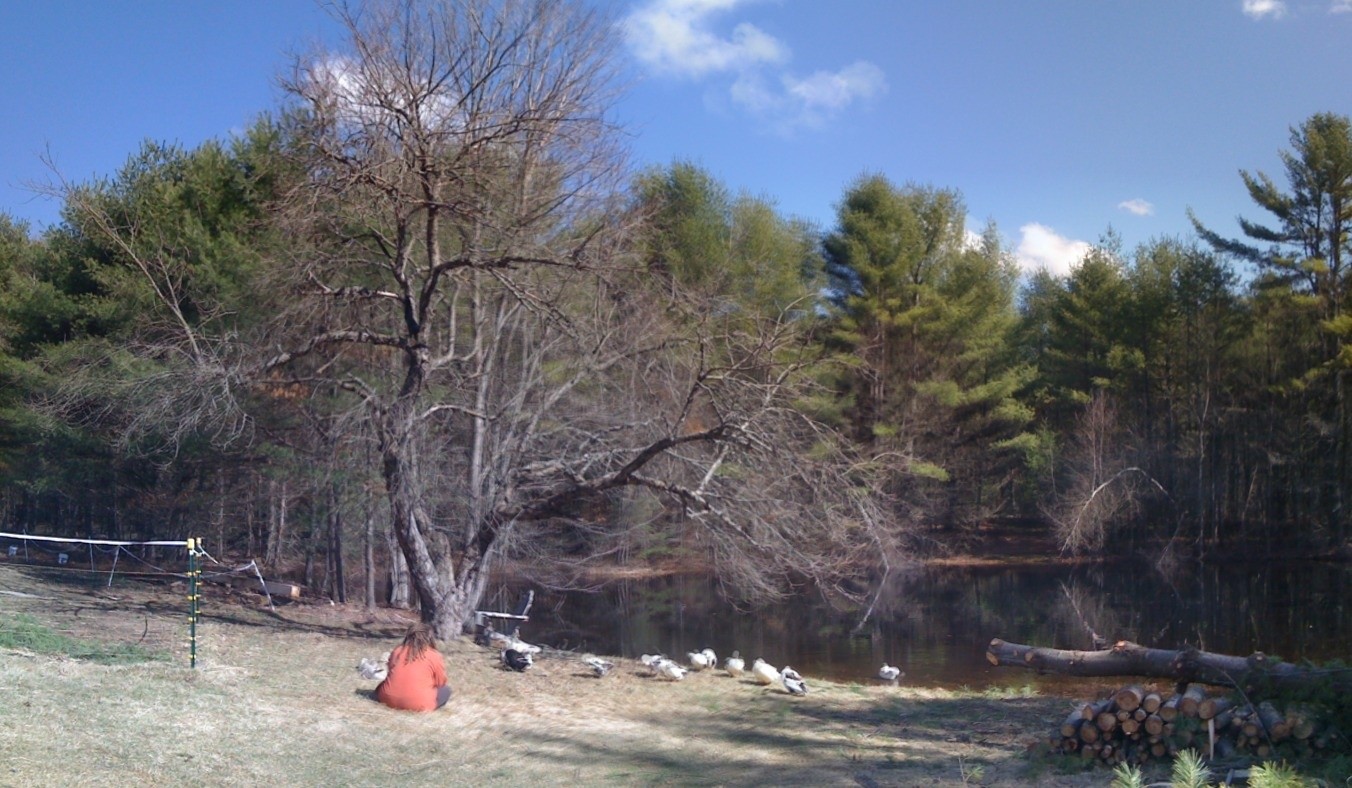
[375,624,453,712]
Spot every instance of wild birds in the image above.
[880,663,899,679]
[355,619,807,700]
